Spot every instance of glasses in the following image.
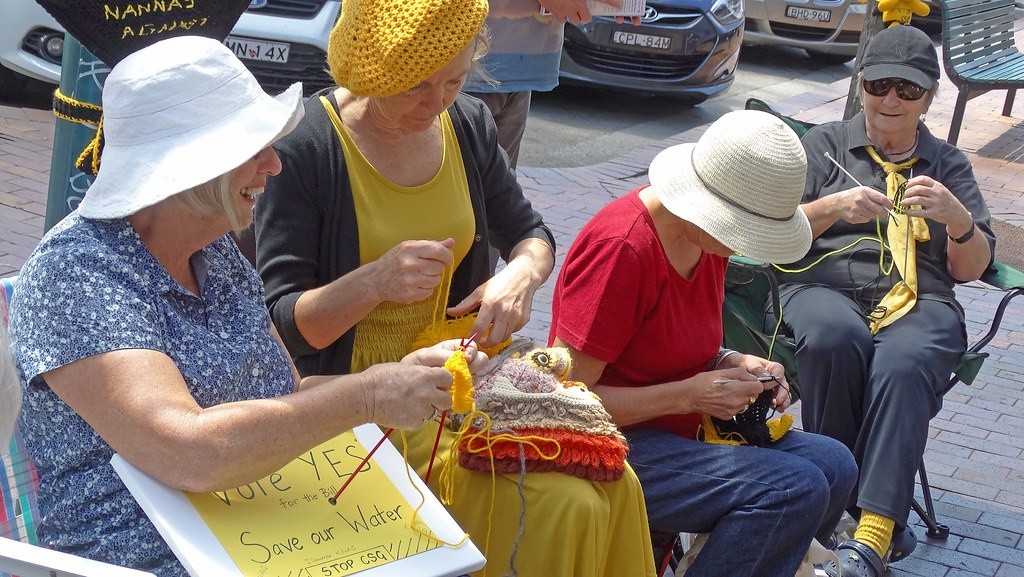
[863,79,926,101]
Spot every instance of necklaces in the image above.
[883,128,918,156]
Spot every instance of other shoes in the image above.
[826,523,917,577]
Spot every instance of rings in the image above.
[925,208,928,219]
[748,396,756,405]
[429,408,438,420]
[739,405,750,414]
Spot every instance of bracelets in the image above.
[946,220,974,244]
[713,349,737,370]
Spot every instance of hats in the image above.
[647,110,813,264]
[328,0,490,95]
[860,23,940,90]
[78,36,305,220]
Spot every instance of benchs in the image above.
[939,0,1024,148]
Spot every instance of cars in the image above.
[0,0,346,113]
[738,0,869,63]
[532,0,745,113]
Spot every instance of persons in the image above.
[460,0,643,170]
[6,35,491,577]
[763,26,998,577]
[254,0,656,577]
[545,110,859,577]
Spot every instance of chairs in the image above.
[720,98,1024,543]
[0,275,157,577]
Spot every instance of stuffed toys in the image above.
[860,0,930,28]
